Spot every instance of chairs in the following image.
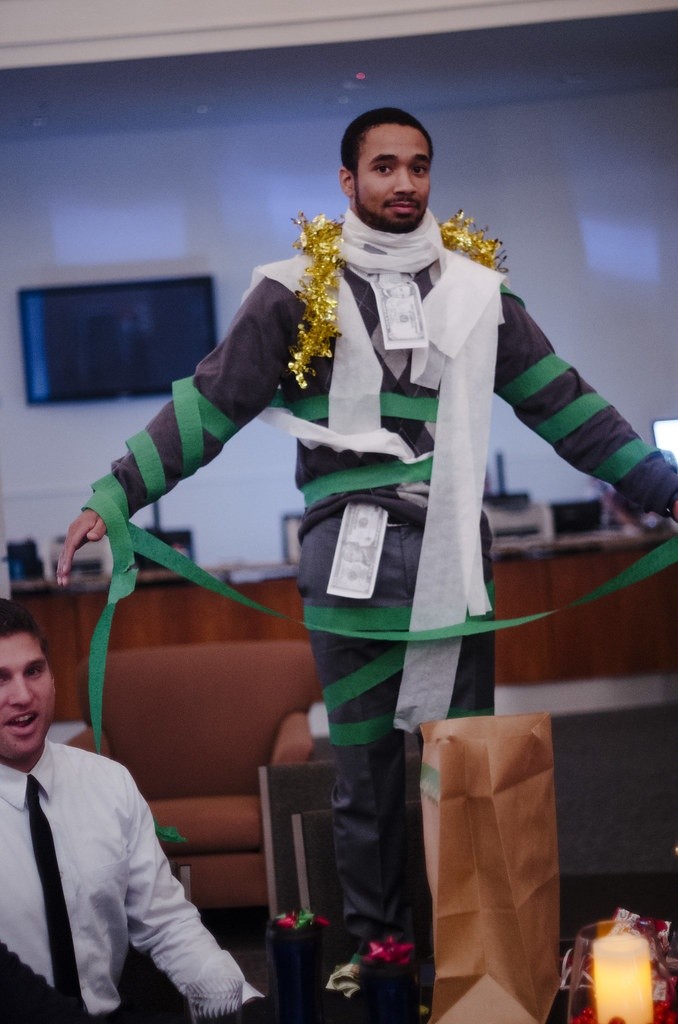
[66,639,322,912]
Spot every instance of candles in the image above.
[593,922,656,1024]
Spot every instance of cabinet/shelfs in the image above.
[0,527,678,722]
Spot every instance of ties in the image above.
[22,773,83,1006]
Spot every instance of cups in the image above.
[185,978,243,1024]
[567,921,671,1024]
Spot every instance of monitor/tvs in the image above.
[16,274,218,408]
[652,418,678,463]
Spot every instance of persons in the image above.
[57,108,678,938]
[0,597,266,1018]
